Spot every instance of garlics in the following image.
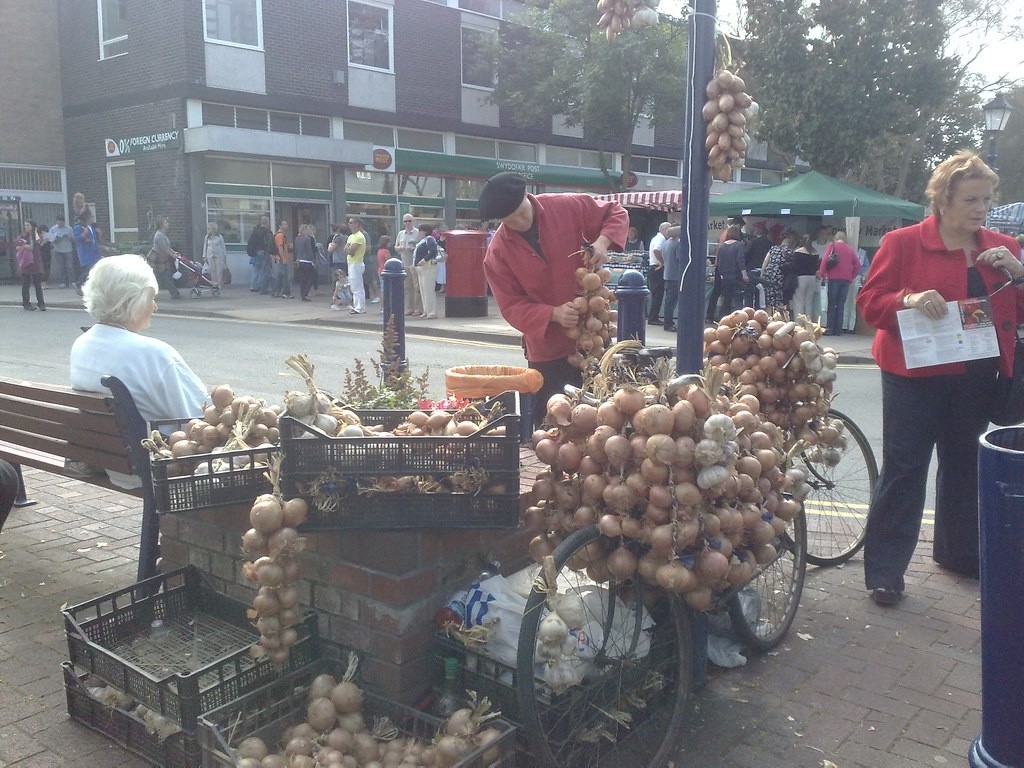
[805,357,822,371]
[703,413,736,442]
[696,440,721,464]
[723,442,739,464]
[696,468,727,487]
[800,341,819,360]
[192,443,275,494]
[816,368,836,385]
[823,346,835,353]
[286,391,364,440]
[821,353,837,369]
[787,418,847,501]
[539,595,585,686]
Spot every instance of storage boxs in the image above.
[147,389,522,532]
[60,561,679,768]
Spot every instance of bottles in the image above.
[430,658,460,745]
[706,271,716,281]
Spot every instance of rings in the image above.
[924,301,931,306]
[996,251,1003,259]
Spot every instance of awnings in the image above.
[591,170,722,213]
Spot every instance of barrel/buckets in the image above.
[968,425,1024,768]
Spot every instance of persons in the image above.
[989,227,1024,266]
[478,173,630,431]
[153,217,185,300]
[855,149,1024,605]
[246,213,496,319]
[625,216,870,336]
[37,193,124,296]
[16,218,47,311]
[202,222,226,297]
[71,254,213,490]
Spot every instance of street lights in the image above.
[982,91,1015,173]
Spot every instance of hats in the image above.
[476,172,526,221]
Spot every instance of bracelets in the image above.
[907,293,913,309]
[1012,275,1024,285]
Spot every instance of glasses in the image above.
[403,220,412,223]
[972,261,1013,297]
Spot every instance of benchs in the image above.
[0,377,205,618]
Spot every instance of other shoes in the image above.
[171,294,185,299]
[946,560,981,578]
[24,304,35,310]
[420,313,437,319]
[58,283,67,287]
[69,283,77,288]
[349,308,367,314]
[648,320,664,325]
[871,588,899,603]
[404,310,422,316]
[666,325,678,332]
[37,304,47,311]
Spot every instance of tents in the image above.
[986,202,1024,232]
[708,170,927,335]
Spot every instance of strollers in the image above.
[170,251,232,299]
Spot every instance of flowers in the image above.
[338,313,470,411]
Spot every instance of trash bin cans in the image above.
[444,364,545,447]
[967,424,1024,768]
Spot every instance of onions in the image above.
[568,268,617,370]
[393,410,506,489]
[596,0,661,43]
[237,674,500,767]
[153,386,283,474]
[243,494,307,665]
[702,69,758,186]
[525,309,838,609]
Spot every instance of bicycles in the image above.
[515,402,879,768]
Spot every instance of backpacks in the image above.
[266,232,287,255]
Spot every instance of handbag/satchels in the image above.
[223,264,231,283]
[826,237,839,270]
[842,329,855,333]
[978,339,1024,427]
[426,236,448,264]
